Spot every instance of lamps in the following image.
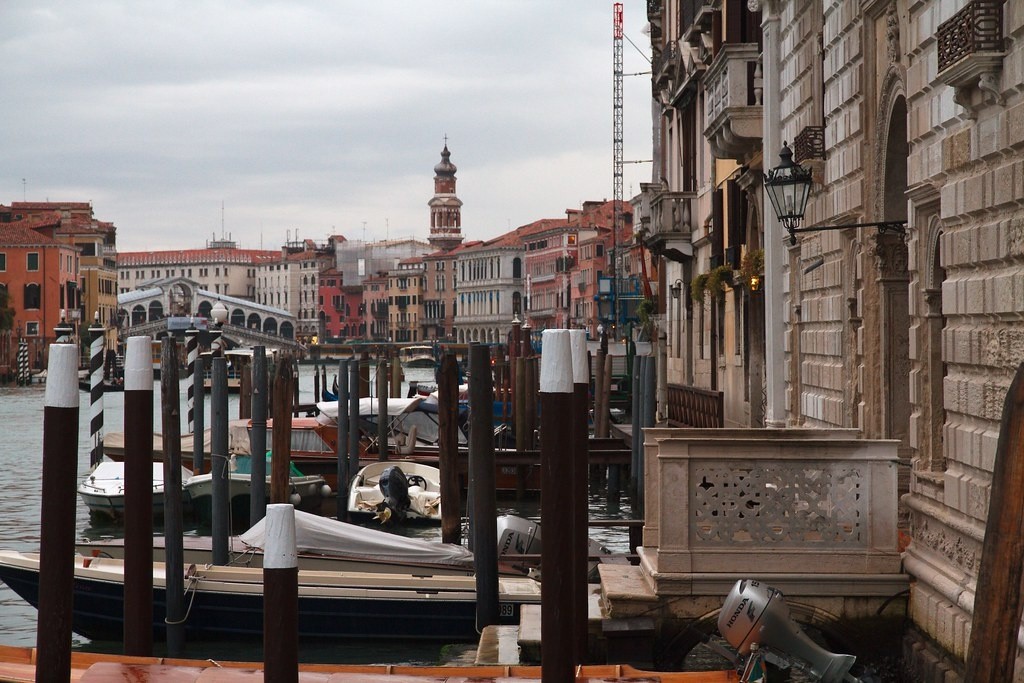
[763,140,907,247]
[670,279,693,299]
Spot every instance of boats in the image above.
[80,373,562,533]
[199,344,304,391]
[0,527,642,648]
